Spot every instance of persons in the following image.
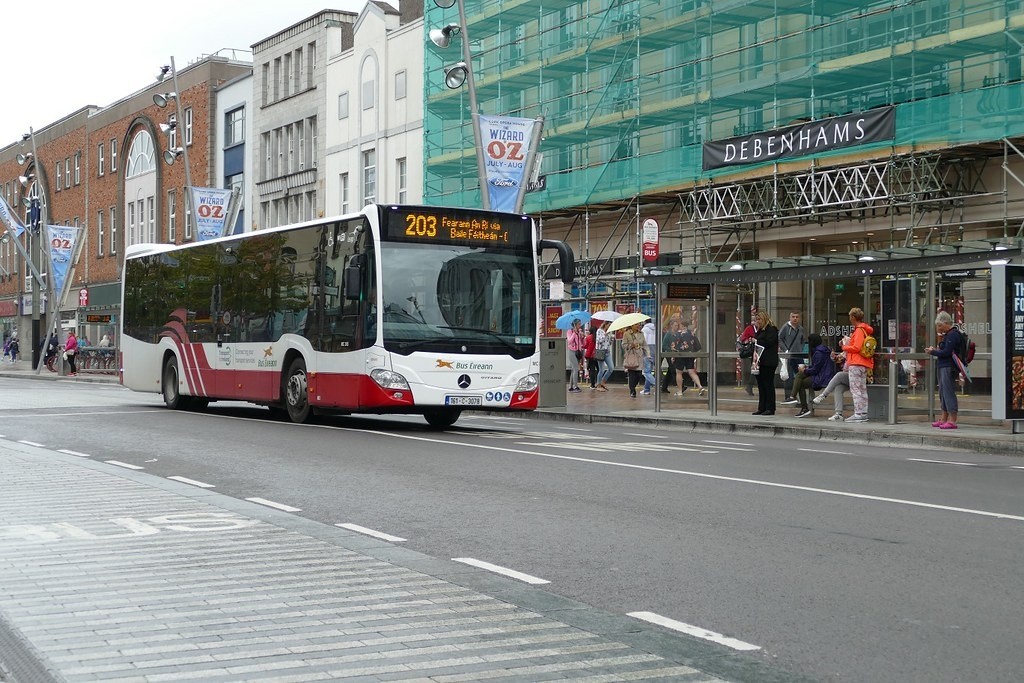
[566,319,585,392]
[660,320,705,396]
[924,311,969,429]
[582,326,599,389]
[640,318,656,396]
[365,284,390,327]
[780,333,835,417]
[813,331,853,421]
[747,310,780,416]
[1,338,19,363]
[95,333,114,369]
[620,323,652,398]
[838,306,875,421]
[778,310,806,400]
[63,331,78,377]
[595,320,614,392]
[739,317,756,396]
[39,333,58,365]
[75,334,92,368]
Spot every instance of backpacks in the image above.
[859,327,876,357]
[952,329,976,364]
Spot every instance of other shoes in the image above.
[682,387,687,392]
[590,385,608,392]
[67,372,77,376]
[674,392,683,396]
[568,387,574,392]
[574,387,581,391]
[631,392,636,397]
[699,387,705,395]
[660,388,670,393]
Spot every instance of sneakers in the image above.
[640,390,650,395]
[813,394,826,404]
[795,408,811,417]
[845,413,862,422]
[745,386,753,395]
[827,413,843,421]
[780,397,798,404]
[860,414,869,421]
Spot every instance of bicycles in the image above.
[47,349,119,373]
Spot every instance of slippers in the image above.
[932,422,943,427]
[940,422,958,429]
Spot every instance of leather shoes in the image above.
[759,410,775,415]
[752,410,763,415]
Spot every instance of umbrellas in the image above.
[939,341,972,383]
[606,313,651,333]
[591,310,623,321]
[555,311,591,329]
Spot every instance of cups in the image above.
[798,364,804,368]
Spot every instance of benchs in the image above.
[809,384,909,421]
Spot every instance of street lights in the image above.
[152,55,199,243]
[428,0,491,209]
[16,125,65,375]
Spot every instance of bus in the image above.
[118,204,576,430]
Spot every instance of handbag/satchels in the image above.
[593,349,605,360]
[623,344,639,368]
[575,350,583,361]
[738,341,755,358]
